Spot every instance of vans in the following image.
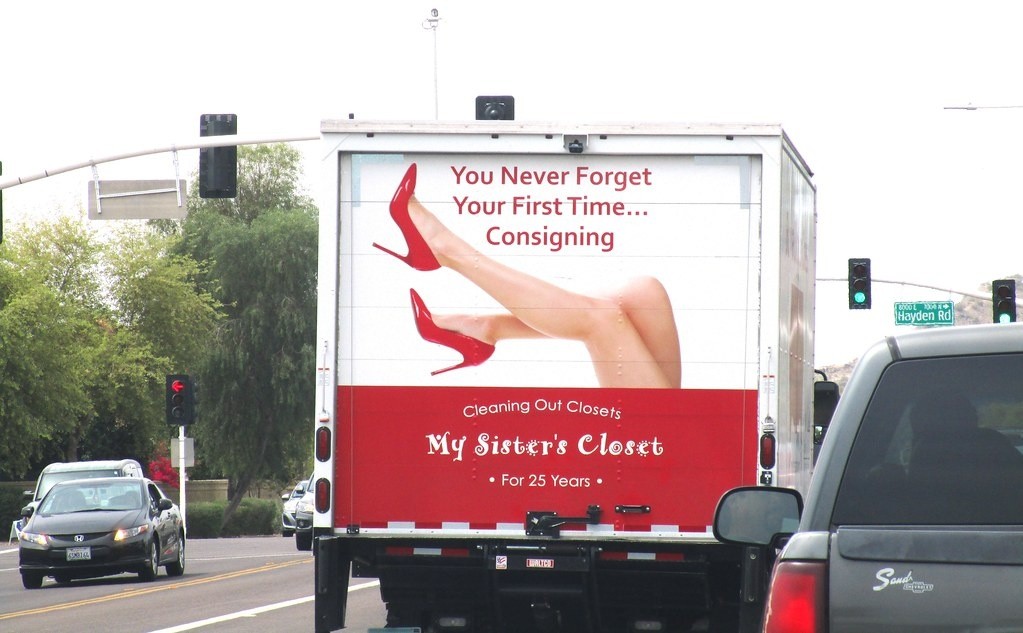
[22,458,146,532]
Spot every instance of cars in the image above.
[17,477,185,589]
[279,473,314,551]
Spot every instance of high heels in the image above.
[372,162,442,272]
[410,288,496,376]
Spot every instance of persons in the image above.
[122,491,140,507]
[371,162,681,388]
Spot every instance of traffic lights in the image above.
[164,374,199,425]
[992,279,1016,323]
[847,258,872,309]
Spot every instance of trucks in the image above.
[312,112,842,633]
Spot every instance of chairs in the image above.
[56,490,87,509]
[947,426,1023,473]
[110,495,126,507]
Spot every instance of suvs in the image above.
[711,323,1023,633]
